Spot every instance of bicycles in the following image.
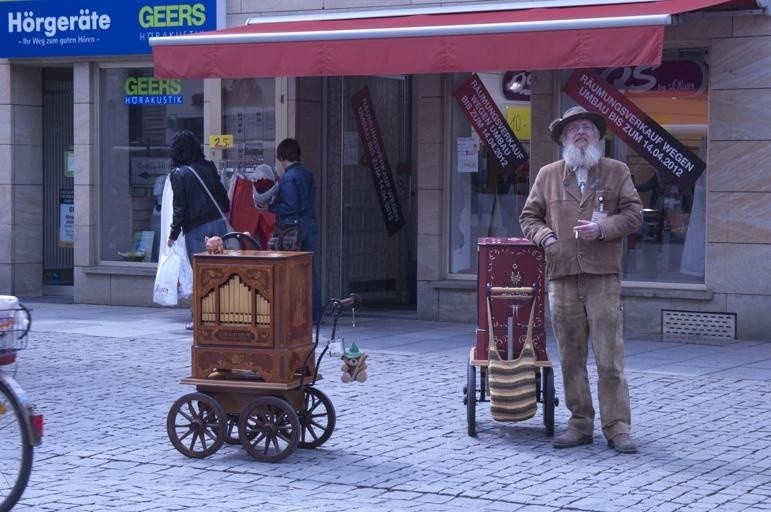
[1,289,44,512]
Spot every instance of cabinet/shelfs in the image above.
[475,236,549,361]
[189,249,316,383]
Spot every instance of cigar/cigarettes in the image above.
[573,230,580,240]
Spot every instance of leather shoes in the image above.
[608,433,638,455]
[552,429,594,450]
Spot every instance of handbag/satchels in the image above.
[224,219,241,250]
[175,240,193,300]
[487,294,538,423]
[152,244,182,306]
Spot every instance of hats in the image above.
[550,104,608,147]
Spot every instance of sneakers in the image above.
[185,322,194,330]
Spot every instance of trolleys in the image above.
[463,237,559,438]
[167,231,362,465]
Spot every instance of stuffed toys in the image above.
[204,234,224,254]
[341,351,369,384]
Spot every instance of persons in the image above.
[166,128,231,331]
[519,104,646,455]
[254,137,325,326]
[138,175,166,263]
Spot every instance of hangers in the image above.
[219,159,230,178]
